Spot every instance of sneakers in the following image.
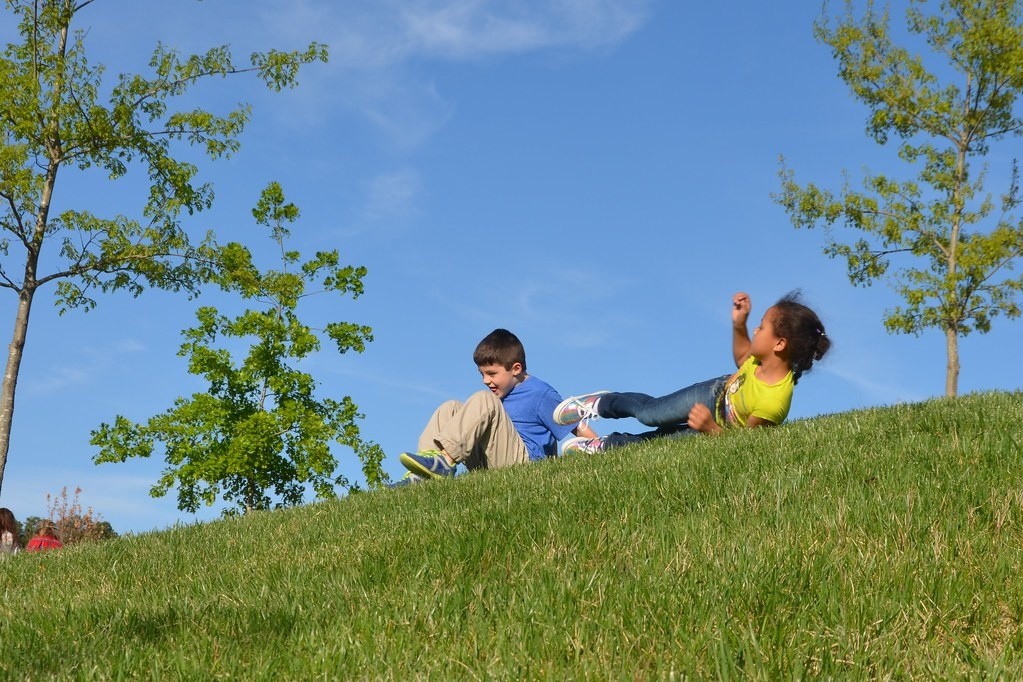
[382,470,423,491]
[400,449,457,479]
[552,391,611,431]
[561,435,608,457]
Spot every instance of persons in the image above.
[0,508,22,554]
[553,292,831,456]
[26,520,62,552]
[382,329,599,491]
